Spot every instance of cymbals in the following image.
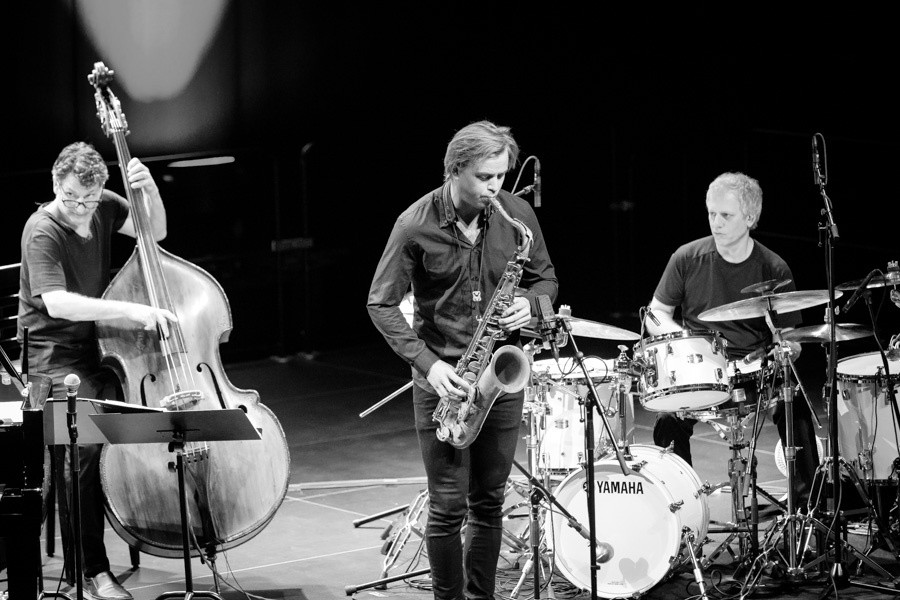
[523,315,642,341]
[836,267,900,290]
[780,322,875,343]
[696,277,845,323]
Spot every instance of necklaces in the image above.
[452,224,487,302]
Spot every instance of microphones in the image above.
[533,293,561,361]
[567,518,614,562]
[744,344,776,364]
[642,305,661,327]
[534,160,541,207]
[812,136,822,185]
[842,269,880,313]
[63,372,83,442]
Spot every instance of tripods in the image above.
[698,244,900,599]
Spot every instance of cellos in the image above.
[79,60,291,597]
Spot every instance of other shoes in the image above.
[805,535,817,550]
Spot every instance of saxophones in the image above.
[433,195,536,449]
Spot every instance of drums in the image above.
[527,355,632,478]
[545,443,709,599]
[675,358,781,420]
[833,351,900,487]
[632,328,734,413]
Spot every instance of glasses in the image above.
[57,180,103,209]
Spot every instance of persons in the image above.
[17,141,179,600]
[644,173,828,519]
[367,120,559,600]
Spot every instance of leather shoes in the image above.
[82,572,134,600]
